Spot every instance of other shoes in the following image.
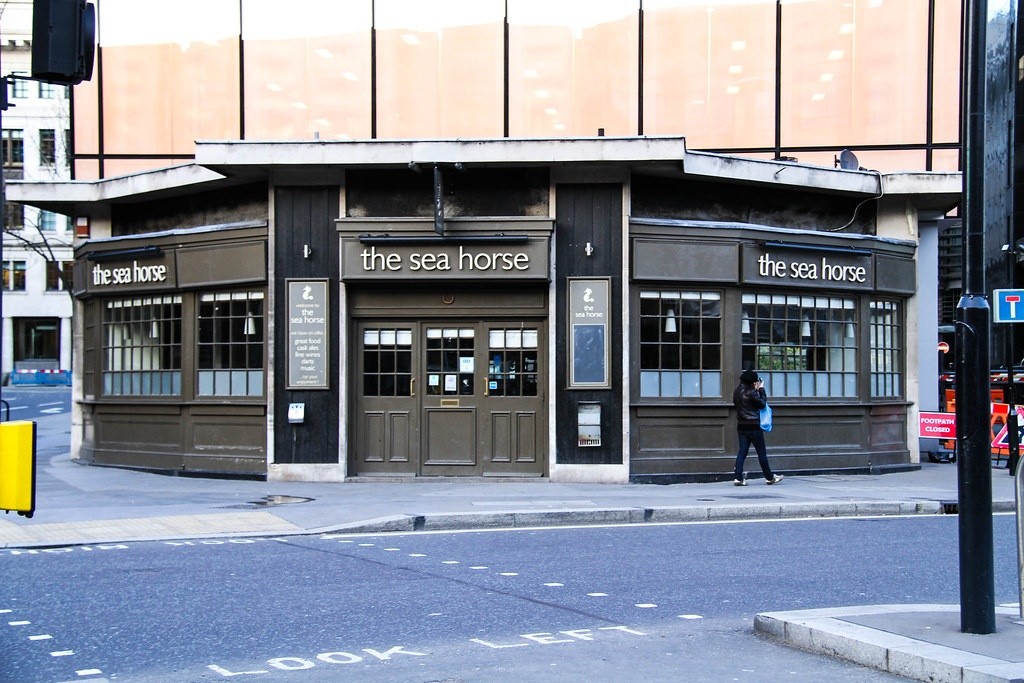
[766,475,784,485]
[734,478,748,486]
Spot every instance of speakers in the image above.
[30,0,95,86]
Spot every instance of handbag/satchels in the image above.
[759,403,773,432]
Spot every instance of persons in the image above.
[732,369,784,486]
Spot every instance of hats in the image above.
[740,370,758,383]
